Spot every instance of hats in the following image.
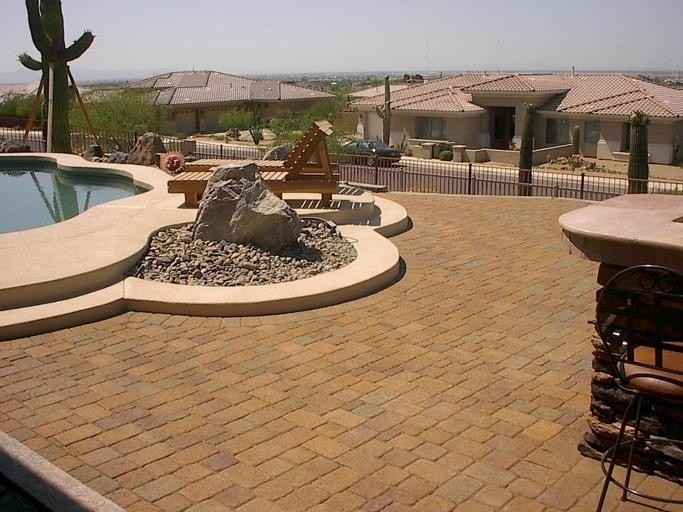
[336,138,401,167]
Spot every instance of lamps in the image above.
[595,265,683,512]
[167,118,340,208]
